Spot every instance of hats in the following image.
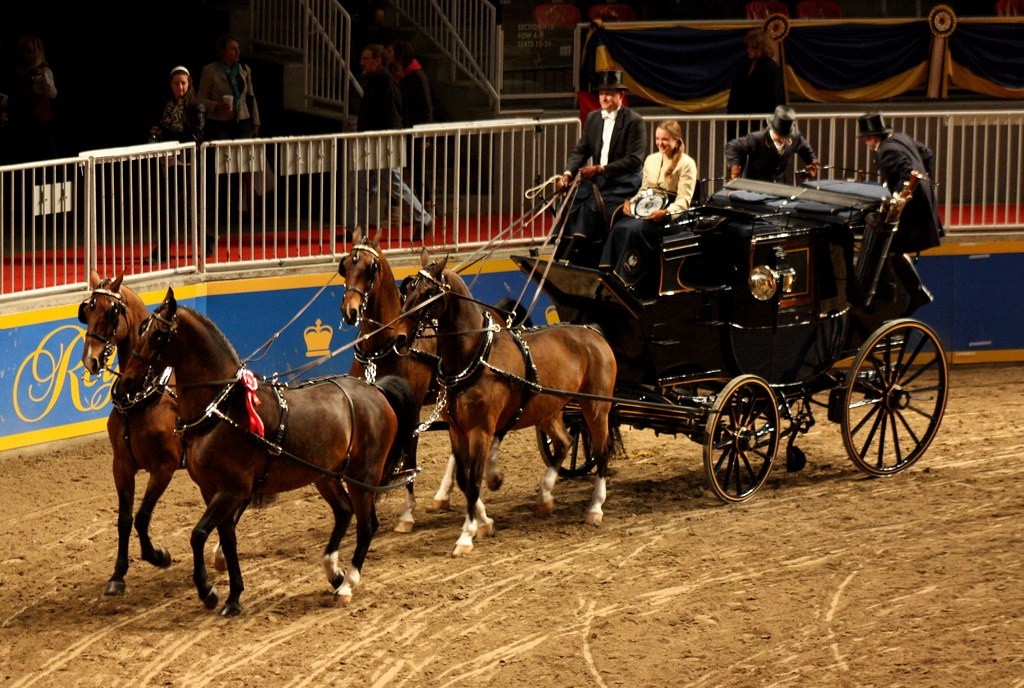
[855,114,892,139]
[768,105,798,137]
[592,70,628,91]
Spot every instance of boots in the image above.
[372,206,401,229]
[424,201,435,219]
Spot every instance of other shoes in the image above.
[407,221,434,242]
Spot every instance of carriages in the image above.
[78,164,949,616]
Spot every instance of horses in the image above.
[339,225,534,534]
[81,265,187,595]
[390,247,618,558]
[113,287,420,617]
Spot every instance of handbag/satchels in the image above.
[241,62,255,119]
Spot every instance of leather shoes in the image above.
[900,285,934,318]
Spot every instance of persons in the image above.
[855,113,944,313]
[346,40,433,241]
[557,70,647,263]
[0,35,57,162]
[725,105,820,184]
[726,27,787,140]
[599,119,697,286]
[139,36,277,264]
